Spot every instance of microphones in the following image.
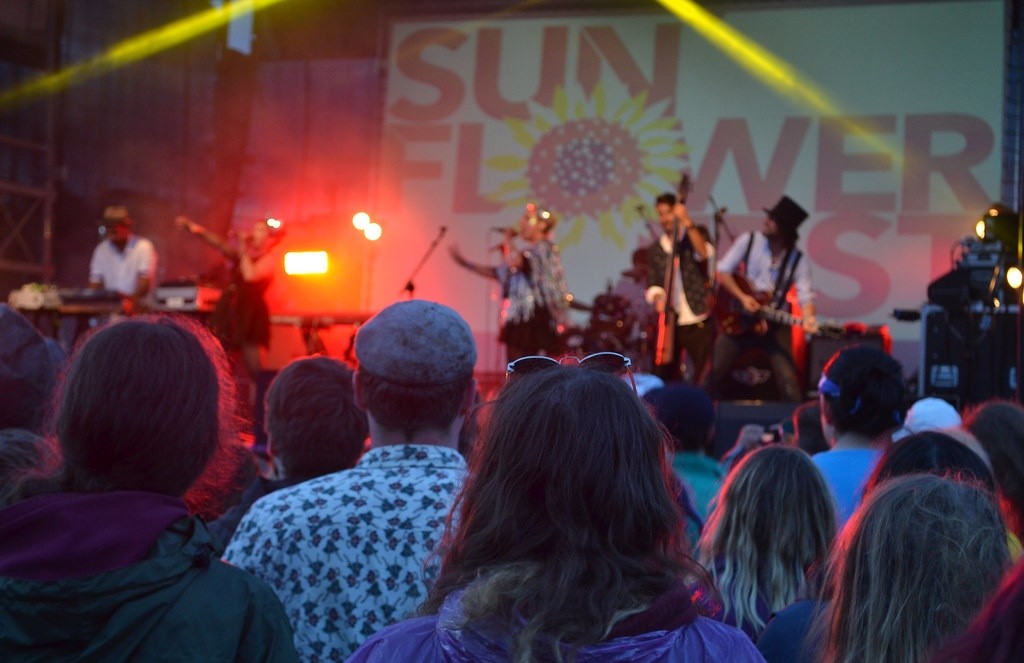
[490,226,518,237]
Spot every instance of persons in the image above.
[628,341,1024,663]
[343,352,768,663]
[174,214,284,402]
[86,207,156,315]
[447,206,567,364]
[698,195,821,401]
[1,299,487,663]
[645,192,714,387]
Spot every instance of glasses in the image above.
[505,352,638,404]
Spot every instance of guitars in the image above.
[652,169,694,366]
[715,272,846,342]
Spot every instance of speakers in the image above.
[808,331,884,397]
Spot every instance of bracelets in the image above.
[685,223,699,232]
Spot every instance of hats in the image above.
[760,194,808,239]
[892,397,962,444]
[93,205,138,227]
[643,385,715,433]
[353,299,478,385]
